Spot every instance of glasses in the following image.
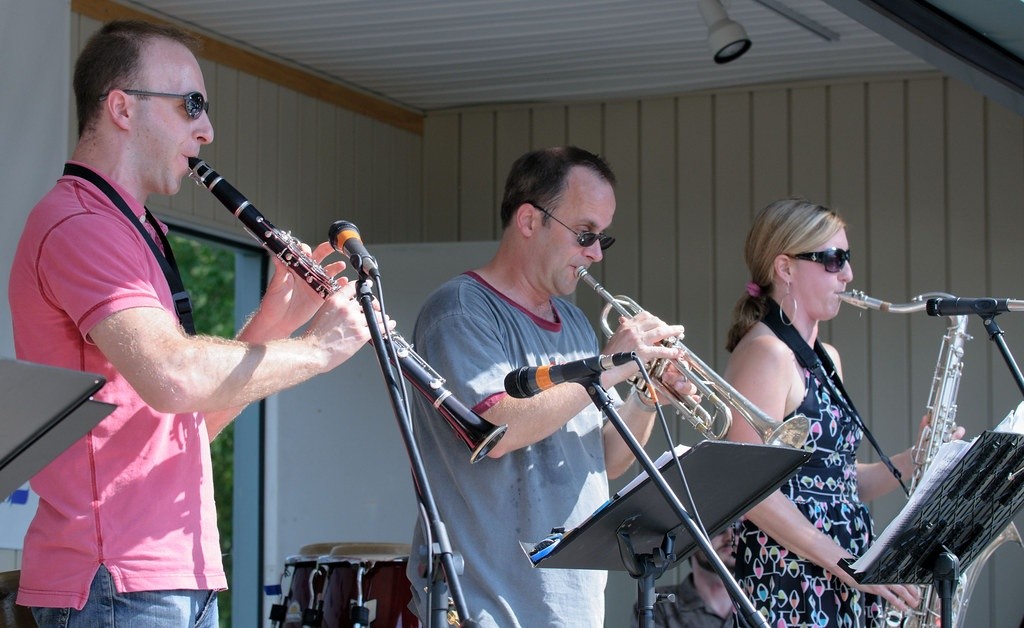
[99,90,211,121]
[784,247,852,273]
[532,205,615,250]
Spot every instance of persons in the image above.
[634,526,750,628]
[719,199,965,628]
[406,146,701,628]
[8,19,397,628]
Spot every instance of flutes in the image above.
[186,155,510,467]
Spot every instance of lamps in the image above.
[697,0,752,64]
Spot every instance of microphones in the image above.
[326,217,382,278]
[501,352,637,400]
[924,296,1024,319]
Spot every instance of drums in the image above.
[274,541,424,628]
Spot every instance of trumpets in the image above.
[572,263,811,452]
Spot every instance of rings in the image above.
[890,584,894,591]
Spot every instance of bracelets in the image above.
[630,385,663,412]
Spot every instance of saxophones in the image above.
[837,288,1024,628]
